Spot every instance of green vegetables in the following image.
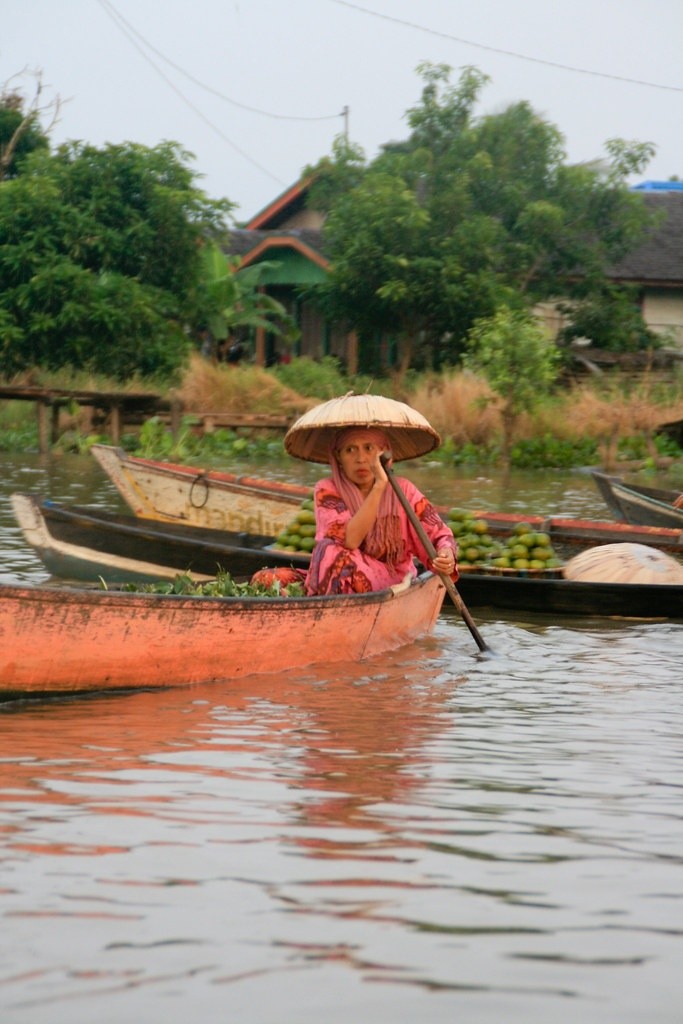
[92,562,305,597]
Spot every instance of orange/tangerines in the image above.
[273,489,317,555]
[442,506,562,568]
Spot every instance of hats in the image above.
[283,392,441,463]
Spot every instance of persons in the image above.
[250,427,461,598]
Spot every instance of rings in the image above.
[448,563,452,567]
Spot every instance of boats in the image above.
[10,490,683,623]
[0,544,449,691]
[592,468,683,530]
[89,443,683,578]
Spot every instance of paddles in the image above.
[374,452,492,652]
